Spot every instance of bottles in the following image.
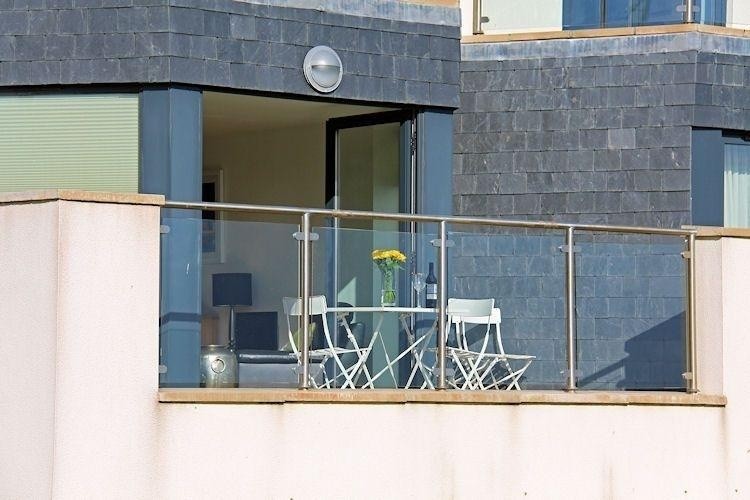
[424,262,439,308]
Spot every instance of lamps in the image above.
[302,43,344,94]
[212,273,253,354]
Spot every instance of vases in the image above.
[378,268,400,306]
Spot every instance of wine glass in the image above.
[413,272,427,308]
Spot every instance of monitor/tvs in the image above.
[235,311,280,352]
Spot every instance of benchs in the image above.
[235,349,325,389]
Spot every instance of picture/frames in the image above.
[202,167,226,265]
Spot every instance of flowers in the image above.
[371,245,407,303]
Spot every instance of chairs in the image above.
[278,295,536,390]
